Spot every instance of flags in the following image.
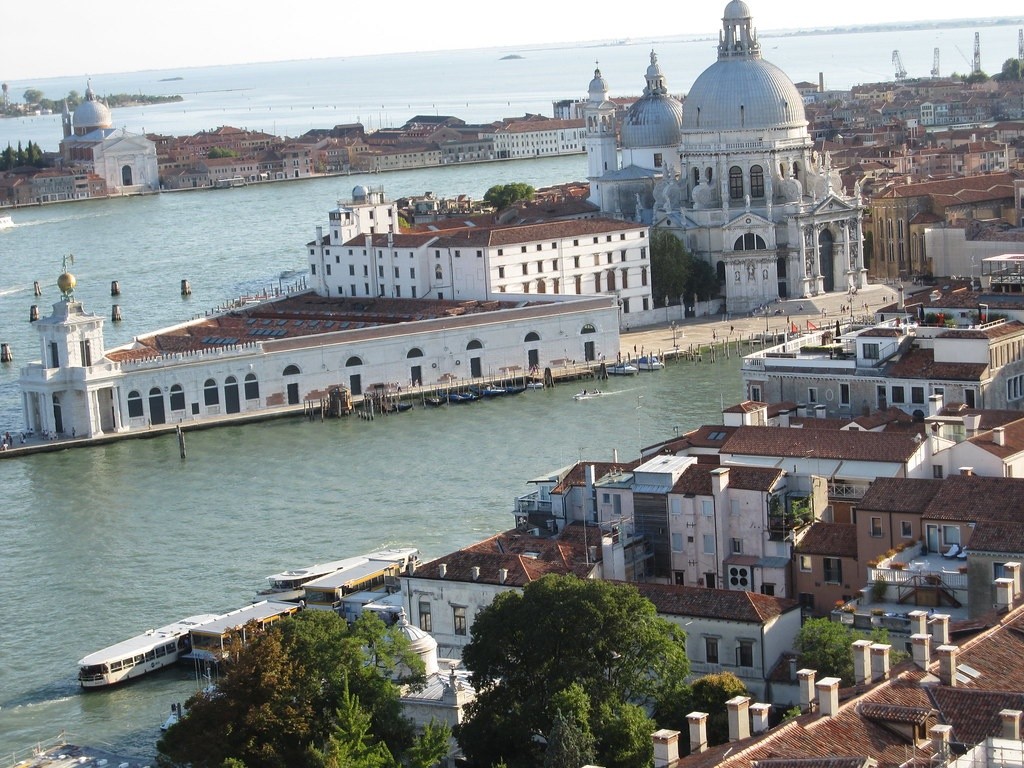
[806,321,816,331]
[791,322,797,334]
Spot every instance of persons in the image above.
[598,352,606,363]
[396,379,420,392]
[713,329,716,340]
[0,426,76,452]
[626,323,629,332]
[750,275,925,318]
[730,325,735,335]
[530,364,540,375]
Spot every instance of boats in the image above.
[421,384,528,407]
[606,365,638,376]
[379,400,412,413]
[636,356,666,371]
[575,391,599,399]
[527,382,545,389]
[77,613,218,687]
[253,550,390,604]
